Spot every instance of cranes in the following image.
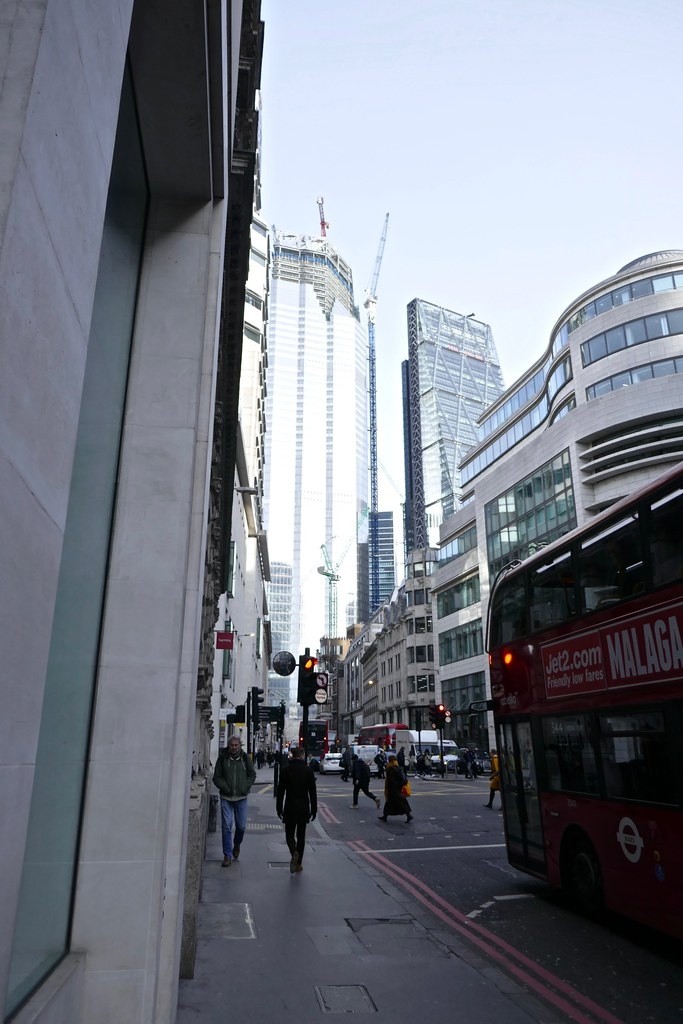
[365,212,389,611]
[318,507,370,654]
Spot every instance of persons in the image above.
[463,745,479,779]
[377,755,414,823]
[397,746,408,776]
[349,754,380,808]
[340,745,351,782]
[212,733,256,867]
[374,748,388,779]
[276,746,317,873]
[257,747,314,770]
[483,747,534,811]
[416,748,433,777]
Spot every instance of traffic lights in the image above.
[428,704,437,729]
[297,655,319,706]
[252,687,264,721]
[437,704,445,728]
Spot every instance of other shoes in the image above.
[405,815,414,824]
[232,842,241,858]
[378,816,388,823]
[296,864,303,874]
[375,798,380,809]
[341,777,349,782]
[290,852,299,873]
[222,857,231,867]
[483,803,492,809]
[349,804,358,809]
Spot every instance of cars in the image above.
[460,748,492,772]
[318,753,345,775]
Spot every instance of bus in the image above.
[470,464,683,941]
[354,722,408,751]
[299,720,329,760]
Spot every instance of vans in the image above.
[437,740,461,771]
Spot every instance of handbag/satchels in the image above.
[339,761,345,768]
[403,783,411,797]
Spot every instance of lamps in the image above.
[422,668,439,673]
[234,487,257,492]
[237,633,255,636]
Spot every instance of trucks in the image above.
[346,745,380,777]
[396,730,442,773]
[288,740,299,753]
